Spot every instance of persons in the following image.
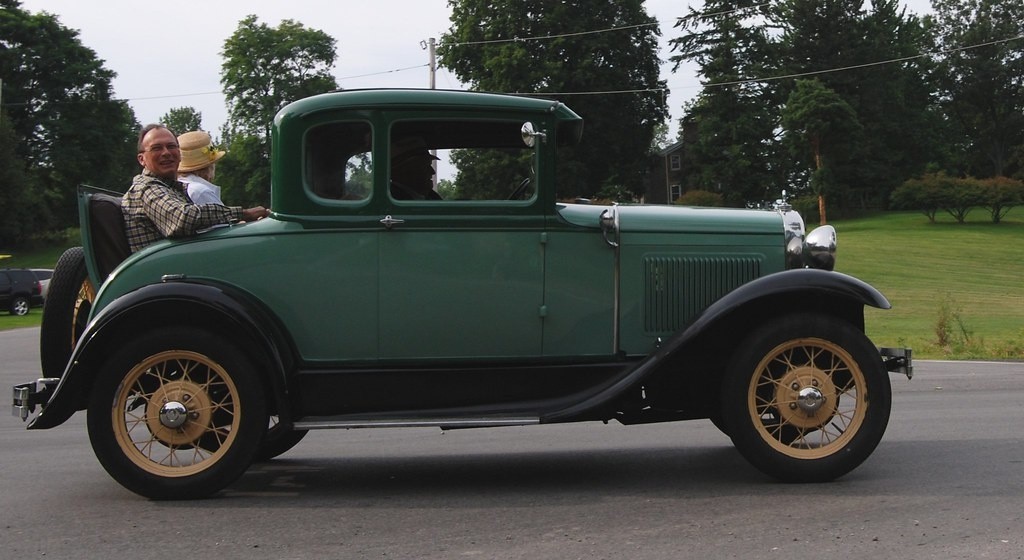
[120,123,268,254]
[177,129,227,205]
[392,136,444,200]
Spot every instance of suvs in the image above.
[30,268,55,301]
[1,269,42,317]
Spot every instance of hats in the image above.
[391,136,440,168]
[177,131,225,172]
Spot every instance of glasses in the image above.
[144,142,178,153]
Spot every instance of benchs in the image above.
[89,191,131,284]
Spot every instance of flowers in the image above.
[201,145,219,161]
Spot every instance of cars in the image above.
[10,86,914,502]
